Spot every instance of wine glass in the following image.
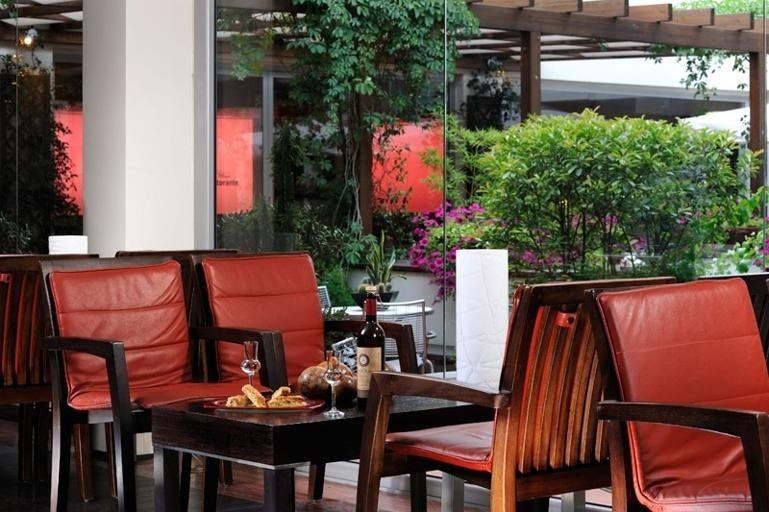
[241,338,260,385]
[322,349,344,417]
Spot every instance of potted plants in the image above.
[349,228,408,312]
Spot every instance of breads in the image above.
[226,396,253,407]
[241,384,268,408]
[271,387,290,398]
[269,396,306,408]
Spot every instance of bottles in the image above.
[356,295,386,410]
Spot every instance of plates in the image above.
[202,396,324,413]
